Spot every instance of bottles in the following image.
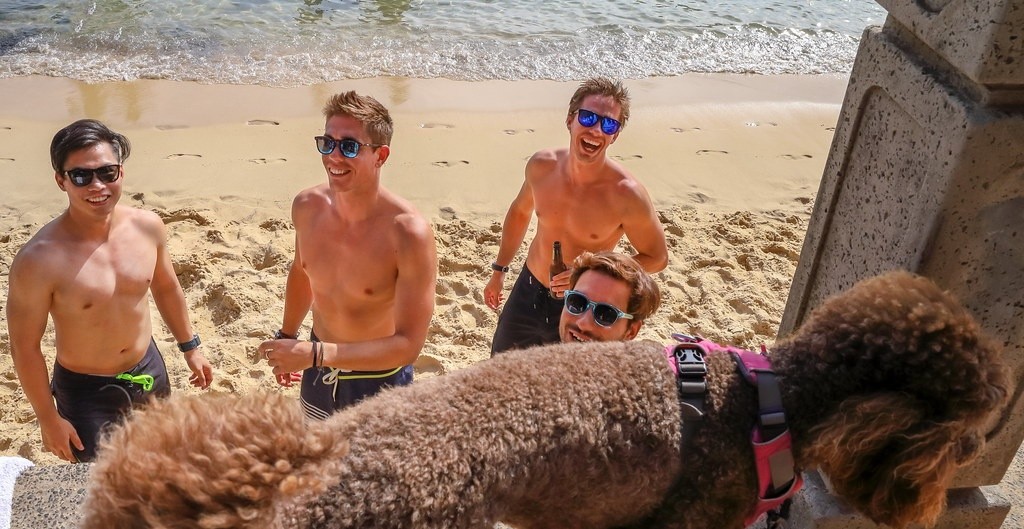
[549,240,567,300]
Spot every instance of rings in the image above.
[266,351,269,360]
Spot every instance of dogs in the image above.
[79,270,1017,529]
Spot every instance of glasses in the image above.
[574,107,621,135]
[563,290,633,329]
[315,135,383,158]
[62,164,121,187]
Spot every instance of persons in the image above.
[484,76,669,358]
[559,249,660,345]
[6,119,212,463]
[258,91,435,429]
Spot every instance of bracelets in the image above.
[319,341,324,369]
[492,263,509,273]
[312,340,317,368]
[178,335,201,353]
[276,331,300,340]
